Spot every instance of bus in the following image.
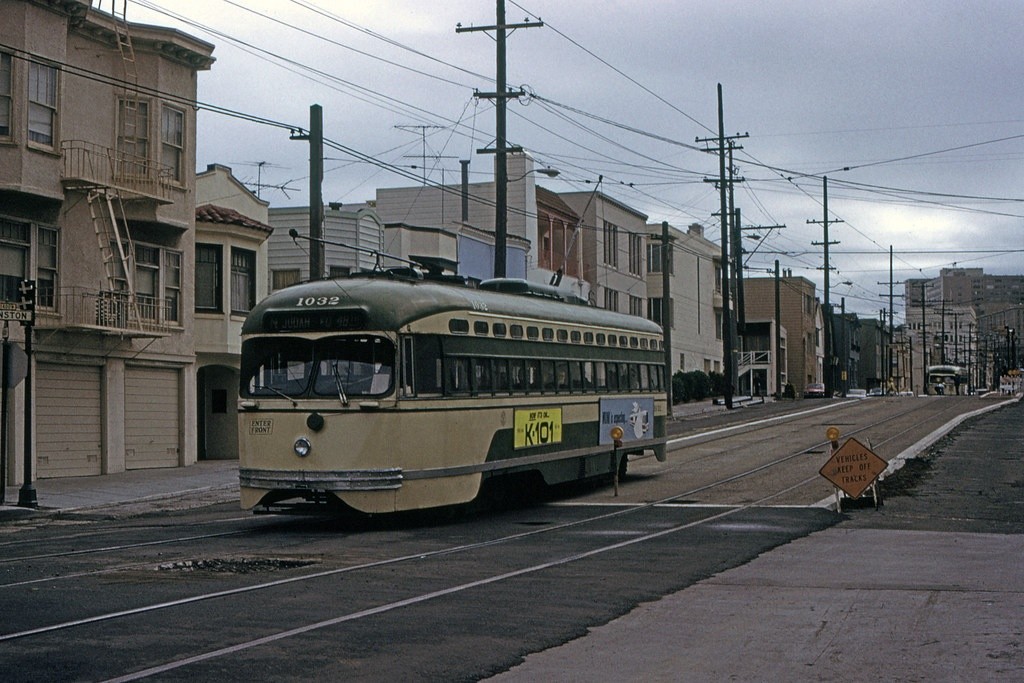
[927,365,968,397]
[241,175,670,531]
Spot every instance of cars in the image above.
[805,382,824,400]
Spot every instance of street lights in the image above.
[493,167,562,280]
[721,234,763,411]
[885,323,910,394]
[822,279,854,398]
